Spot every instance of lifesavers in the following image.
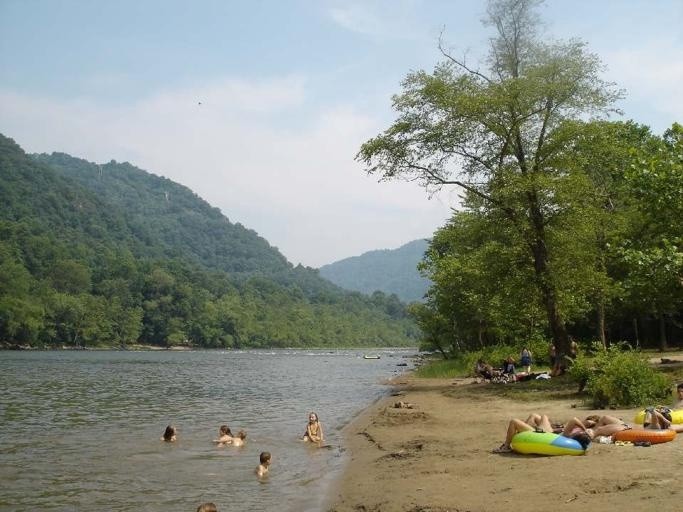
[612,426,676,444]
[512,429,593,455]
[636,407,683,425]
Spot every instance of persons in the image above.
[502,356,516,375]
[217,424,233,448]
[548,336,577,377]
[670,383,683,410]
[490,406,682,455]
[475,357,493,380]
[302,412,325,443]
[212,429,247,448]
[519,346,531,374]
[158,424,177,442]
[253,450,273,484]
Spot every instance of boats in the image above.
[363,354,382,360]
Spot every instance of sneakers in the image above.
[493,444,512,453]
